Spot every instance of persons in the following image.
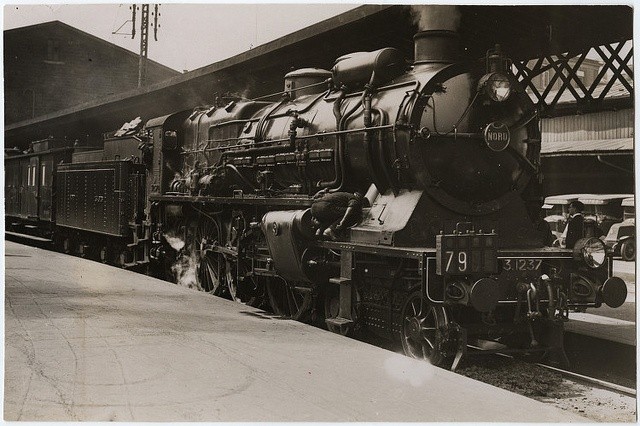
[560,201,585,248]
[311,192,365,240]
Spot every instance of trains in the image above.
[6,29,628,370]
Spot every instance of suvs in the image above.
[603,205,635,263]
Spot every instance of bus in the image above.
[579,190,619,249]
[541,190,576,249]
[622,189,634,250]
